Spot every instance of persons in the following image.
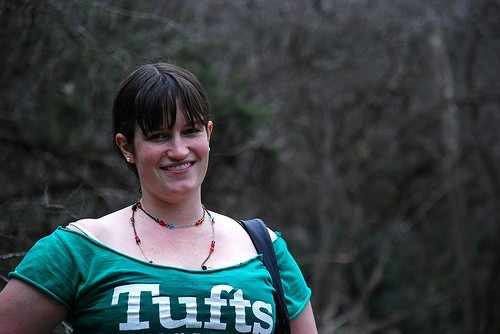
[1,62,319,334]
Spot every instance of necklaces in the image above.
[129,200,217,271]
[138,200,208,229]
